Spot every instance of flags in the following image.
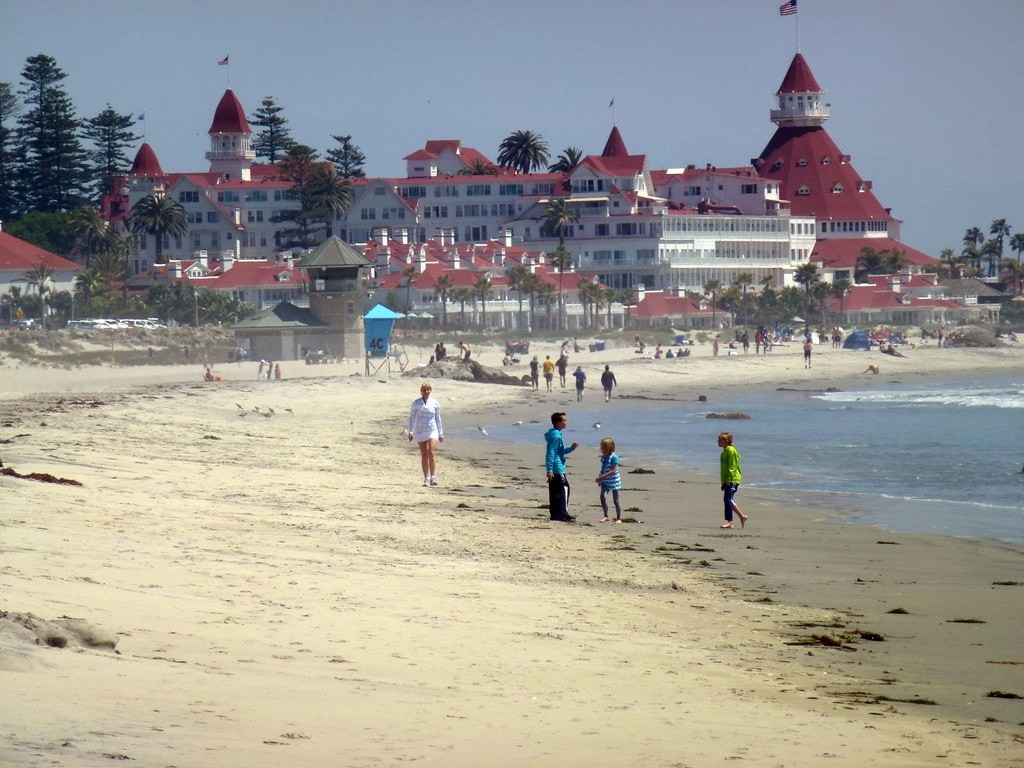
[609,99,614,106]
[218,56,228,65]
[137,114,144,120]
[780,0,798,15]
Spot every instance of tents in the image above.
[843,330,870,350]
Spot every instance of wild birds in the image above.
[235,403,243,410]
[239,413,247,419]
[512,418,523,426]
[481,428,487,435]
[250,406,295,419]
[595,424,601,429]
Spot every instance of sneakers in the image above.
[430,478,438,486]
[423,478,430,486]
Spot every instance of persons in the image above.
[864,324,941,375]
[502,352,513,365]
[258,360,281,379]
[544,413,579,521]
[717,433,748,528]
[573,366,586,401]
[409,383,444,486]
[203,367,214,382]
[560,341,569,358]
[601,366,617,402]
[712,324,842,370]
[654,344,691,358]
[556,354,567,387]
[530,356,539,390]
[429,343,446,363]
[595,438,622,523]
[994,329,1020,342]
[184,346,188,358]
[459,341,471,358]
[543,355,554,392]
